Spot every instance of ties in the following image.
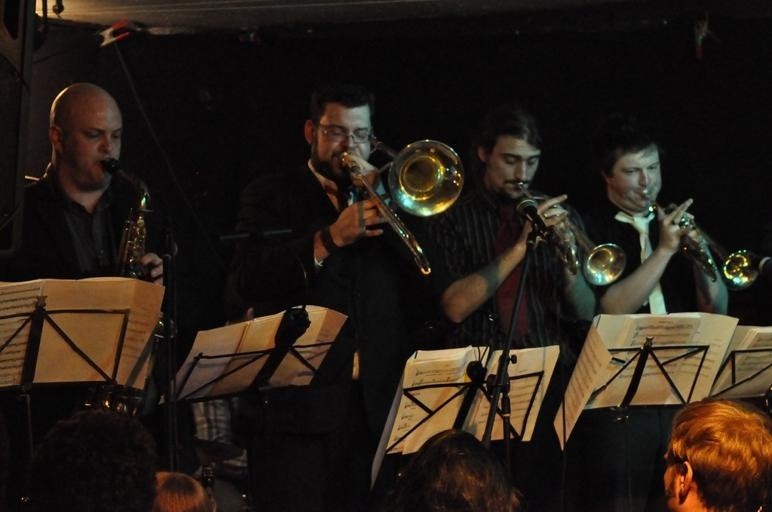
[494,204,529,340]
[614,211,667,316]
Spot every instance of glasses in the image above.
[317,125,377,143]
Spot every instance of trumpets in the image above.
[643,193,764,291]
[341,138,466,276]
[518,182,627,285]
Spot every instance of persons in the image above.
[387,427,517,511]
[658,395,772,512]
[580,114,730,512]
[22,408,154,511]
[23,80,176,423]
[228,78,417,511]
[155,470,217,511]
[420,101,596,511]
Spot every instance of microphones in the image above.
[513,197,555,250]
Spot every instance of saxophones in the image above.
[85,158,169,420]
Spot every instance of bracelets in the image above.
[318,225,342,255]
